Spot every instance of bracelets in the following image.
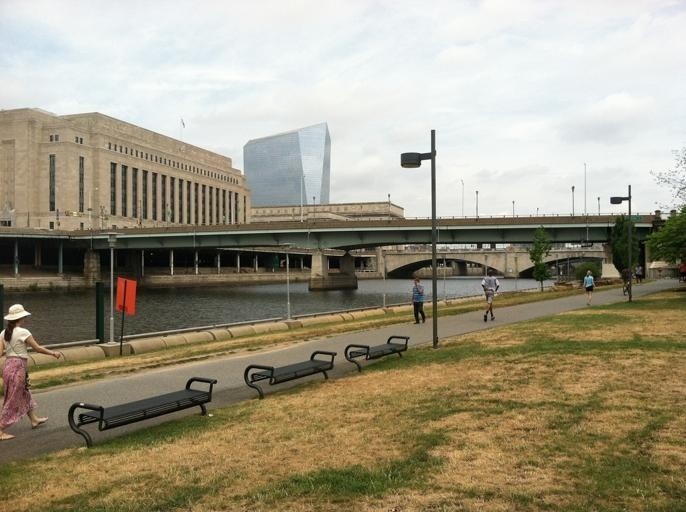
[53,351,55,356]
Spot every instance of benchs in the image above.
[242,349,340,404]
[342,327,416,379]
[66,375,223,450]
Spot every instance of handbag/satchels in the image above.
[26,372,32,389]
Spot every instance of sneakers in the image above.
[484,314,487,321]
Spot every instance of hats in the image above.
[4,303,31,320]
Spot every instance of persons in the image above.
[584,271,598,306]
[0,303,61,442]
[480,268,499,323]
[408,277,425,326]
[621,260,686,296]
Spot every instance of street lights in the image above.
[401,129,441,350]
[554,253,584,285]
[514,256,519,290]
[312,184,603,221]
[280,242,296,323]
[610,184,632,300]
[99,231,123,343]
[88,187,99,229]
[483,254,488,277]
[381,249,388,307]
[440,252,447,297]
